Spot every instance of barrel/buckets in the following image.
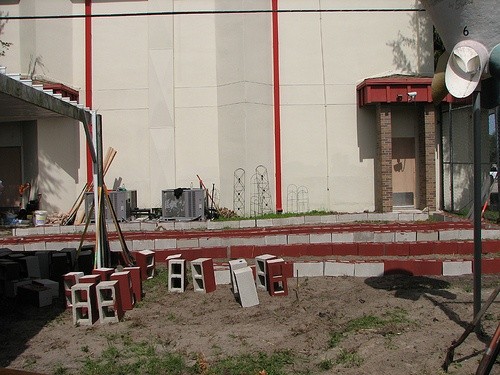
[32,211,48,227]
